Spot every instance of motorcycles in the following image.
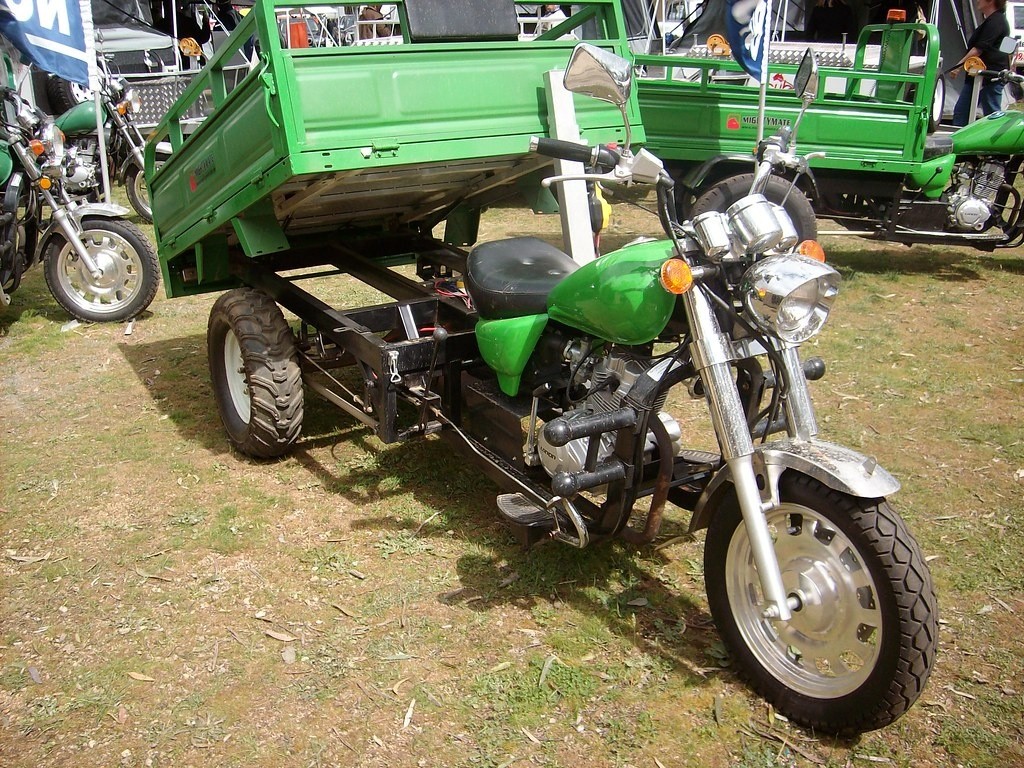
[0,0,1024,737]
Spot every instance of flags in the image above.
[0,0,98,91]
[727,0,772,85]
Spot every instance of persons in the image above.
[541,5,566,34]
[151,0,211,71]
[359,5,392,40]
[952,0,1010,127]
